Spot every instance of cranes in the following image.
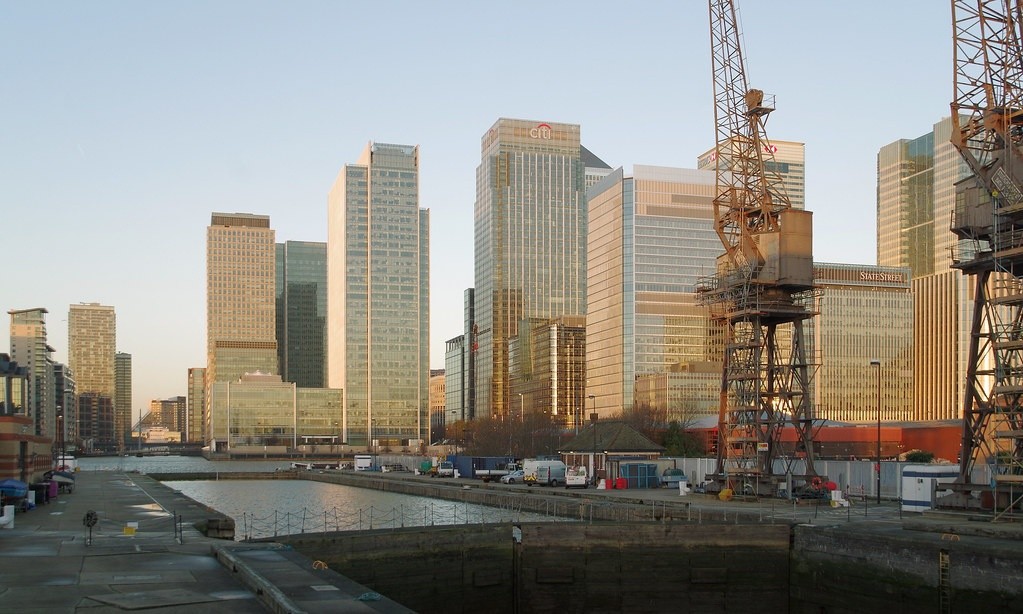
[929,0,1023,518]
[705,0,837,507]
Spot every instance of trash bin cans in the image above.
[0,479,60,529]
[415,468,420,476]
[454,468,460,478]
[831,491,842,507]
[596,478,627,490]
[382,466,390,473]
[679,481,691,495]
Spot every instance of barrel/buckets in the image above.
[616,478,627,489]
[605,479,613,490]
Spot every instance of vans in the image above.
[436,460,455,477]
[522,459,569,487]
[563,466,589,488]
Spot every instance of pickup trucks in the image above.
[659,468,689,489]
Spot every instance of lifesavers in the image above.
[873,464,881,474]
[811,476,822,491]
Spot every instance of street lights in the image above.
[452,409,457,456]
[589,395,597,486]
[334,423,339,466]
[372,418,376,471]
[518,393,524,422]
[870,361,881,507]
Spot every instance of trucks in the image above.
[475,462,521,483]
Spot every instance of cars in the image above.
[500,469,524,484]
